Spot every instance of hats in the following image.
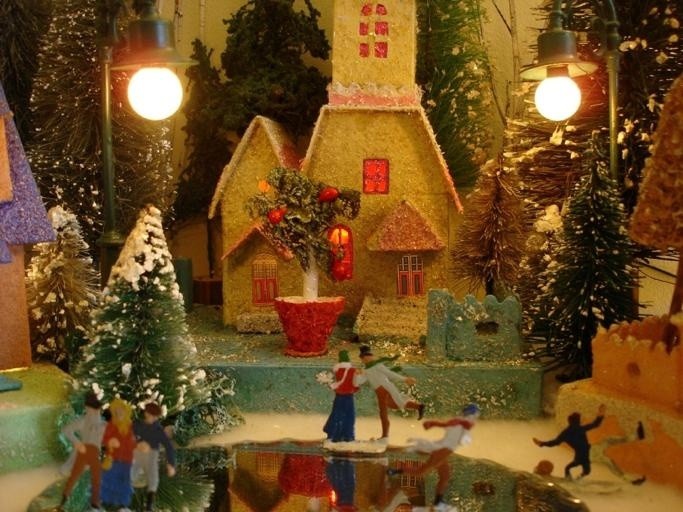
[360,345,374,358]
